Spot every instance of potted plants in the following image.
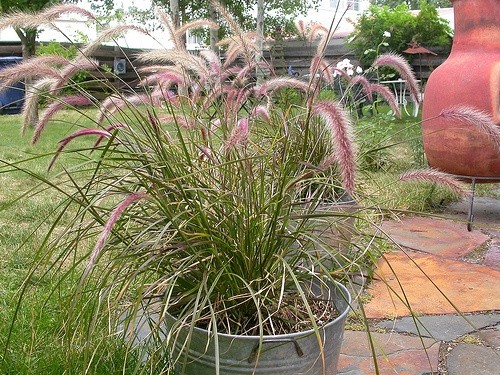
[3,0,494,375]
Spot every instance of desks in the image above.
[379,77,421,118]
